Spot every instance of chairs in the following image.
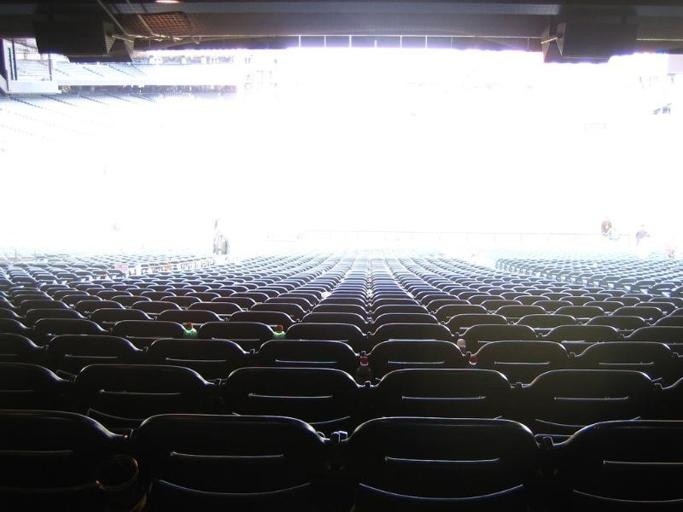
[1,249,683,512]
